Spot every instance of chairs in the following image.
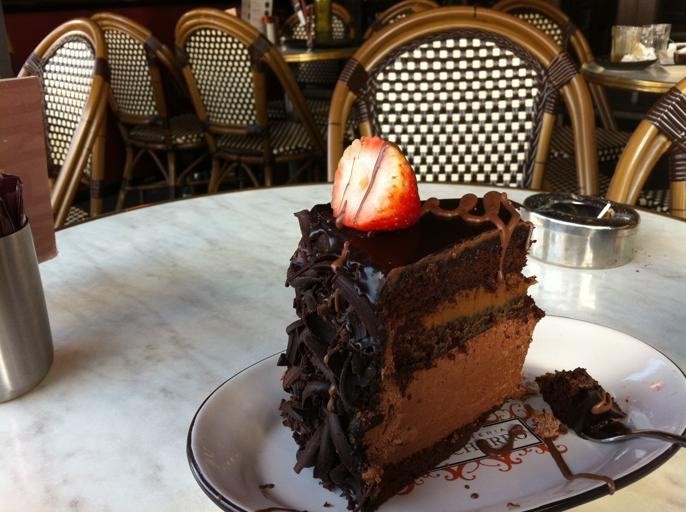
[0,0,685,230]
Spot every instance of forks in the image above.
[576,416,686,449]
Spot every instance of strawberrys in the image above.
[330,136,422,232]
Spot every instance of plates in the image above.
[187,312,685,512]
[595,50,657,70]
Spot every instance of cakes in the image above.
[535,364,627,434]
[276,191,546,512]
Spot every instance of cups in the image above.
[313,0,333,36]
[610,22,672,63]
[264,22,282,50]
[0,216,56,406]
